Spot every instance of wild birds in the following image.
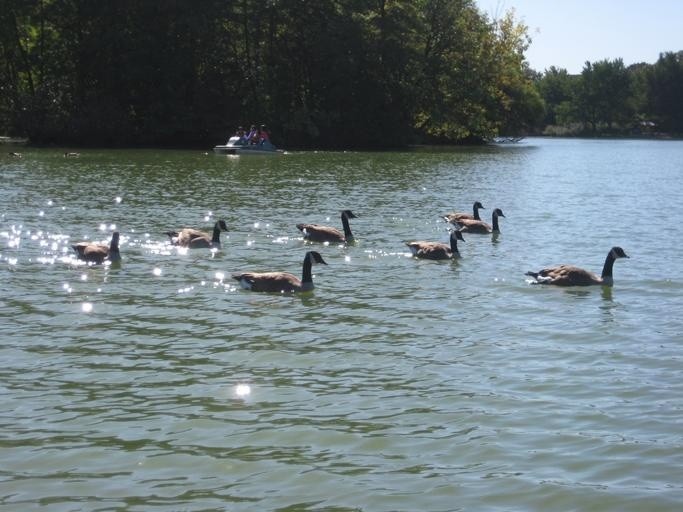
[439,202,485,224]
[524,247,630,286]
[405,229,465,260]
[447,208,506,234]
[163,220,229,248]
[297,210,358,243]
[70,232,122,265]
[232,251,328,292]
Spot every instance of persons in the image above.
[234,126,248,145]
[245,124,259,146]
[257,124,270,146]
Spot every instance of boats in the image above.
[212,136,286,156]
